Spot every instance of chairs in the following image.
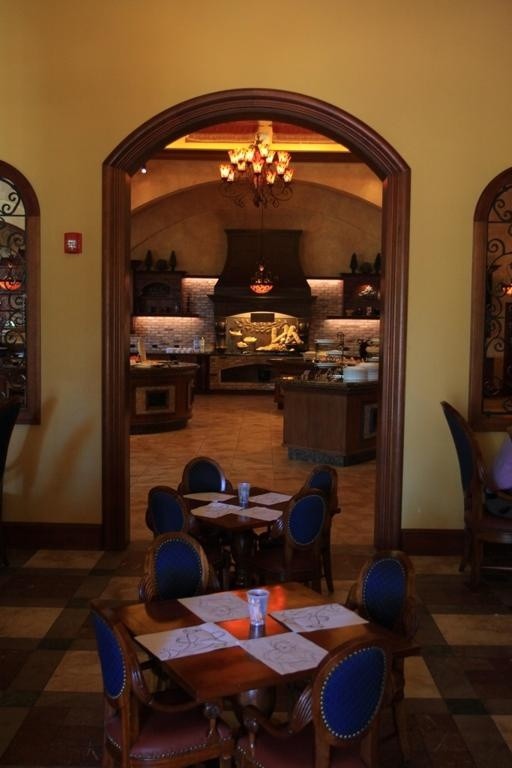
[176,456,234,493]
[336,547,419,667]
[288,465,345,590]
[140,485,229,591]
[259,486,333,595]
[91,596,236,768]
[233,632,395,768]
[133,529,217,678]
[440,401,512,593]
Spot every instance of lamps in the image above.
[217,120,295,208]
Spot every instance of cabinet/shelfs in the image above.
[133,270,187,315]
[340,273,381,316]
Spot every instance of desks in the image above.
[280,374,378,468]
[166,483,342,571]
[114,580,379,765]
[207,351,304,391]
[129,360,200,434]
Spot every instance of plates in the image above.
[316,362,338,369]
[314,338,341,346]
[343,362,379,382]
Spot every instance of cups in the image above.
[246,589,269,626]
[166,348,193,354]
[238,482,250,503]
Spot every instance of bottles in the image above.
[194,336,204,352]
